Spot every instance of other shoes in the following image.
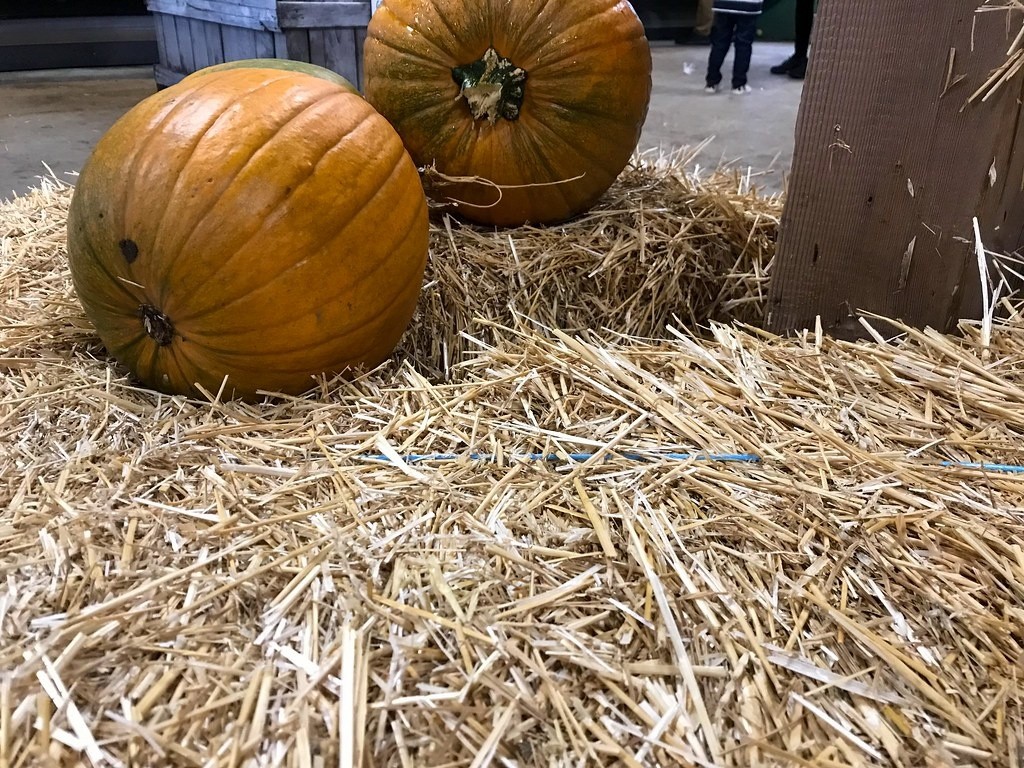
[705,85,718,94]
[732,86,751,94]
[771,54,808,80]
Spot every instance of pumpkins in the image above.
[67,58,429,403]
[364,0,652,228]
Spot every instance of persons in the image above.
[704,0,764,94]
[771,0,819,78]
